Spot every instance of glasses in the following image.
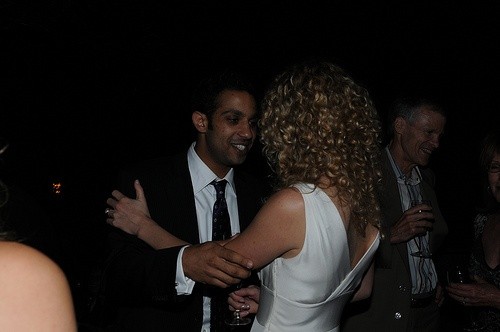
[485,162,499,174]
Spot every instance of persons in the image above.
[0,241,78,332]
[340,101,450,332]
[100,82,270,332]
[105,62,381,332]
[446,132,500,332]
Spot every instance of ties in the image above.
[410,295,428,310]
[209,180,233,332]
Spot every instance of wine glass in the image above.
[222,234,252,326]
[410,201,437,258]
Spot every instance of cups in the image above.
[446,266,488,331]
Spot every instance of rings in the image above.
[105,208,110,215]
[462,297,466,305]
